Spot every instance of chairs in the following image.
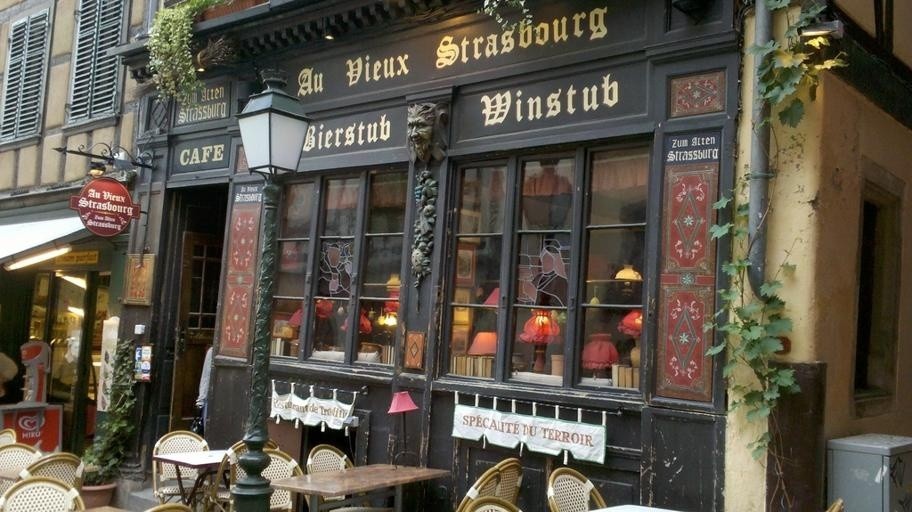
[0,427,87,511]
[144,428,612,512]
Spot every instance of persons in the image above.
[196,346,213,437]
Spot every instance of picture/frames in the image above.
[452,207,483,356]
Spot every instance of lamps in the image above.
[517,258,643,382]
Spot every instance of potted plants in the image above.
[78,336,139,510]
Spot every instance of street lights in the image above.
[235,68,314,509]
